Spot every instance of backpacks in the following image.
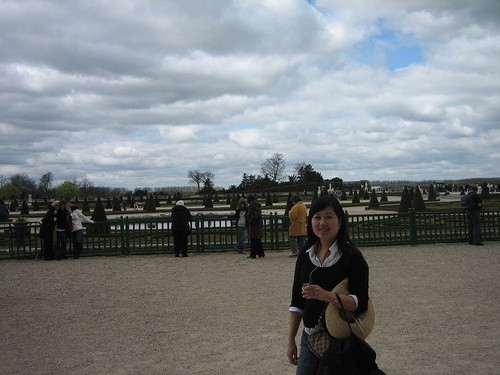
[461,193,476,207]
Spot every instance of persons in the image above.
[284,194,294,216]
[222,197,248,254]
[244,195,265,259]
[53,200,73,259]
[170,200,193,257]
[70,206,97,259]
[287,194,369,375]
[36,200,60,260]
[465,186,485,246]
[288,195,308,257]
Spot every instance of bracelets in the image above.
[331,293,337,304]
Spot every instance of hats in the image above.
[237,197,248,209]
[324,275,375,341]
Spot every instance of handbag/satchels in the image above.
[306,323,339,360]
